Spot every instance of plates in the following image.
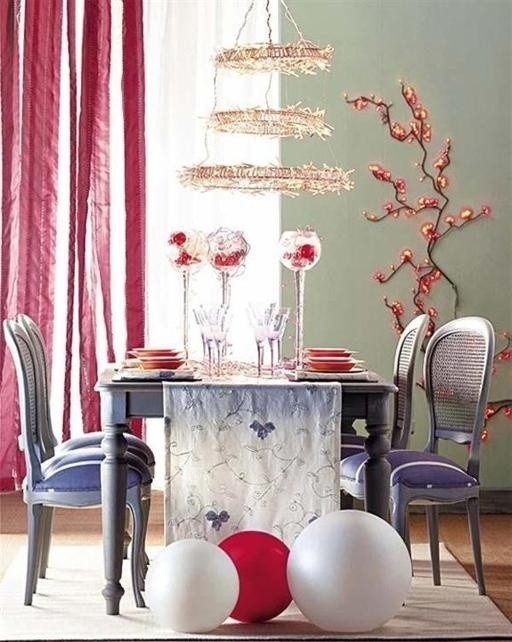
[303,346,357,371]
[127,345,184,370]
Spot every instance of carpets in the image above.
[0,524,511,640]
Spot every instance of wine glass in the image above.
[192,299,235,382]
[245,300,291,380]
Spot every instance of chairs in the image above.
[342,314,497,602]
[0,316,153,610]
[12,309,158,581]
[341,312,431,465]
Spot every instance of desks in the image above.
[92,348,402,619]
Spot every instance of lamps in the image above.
[168,1,358,196]
